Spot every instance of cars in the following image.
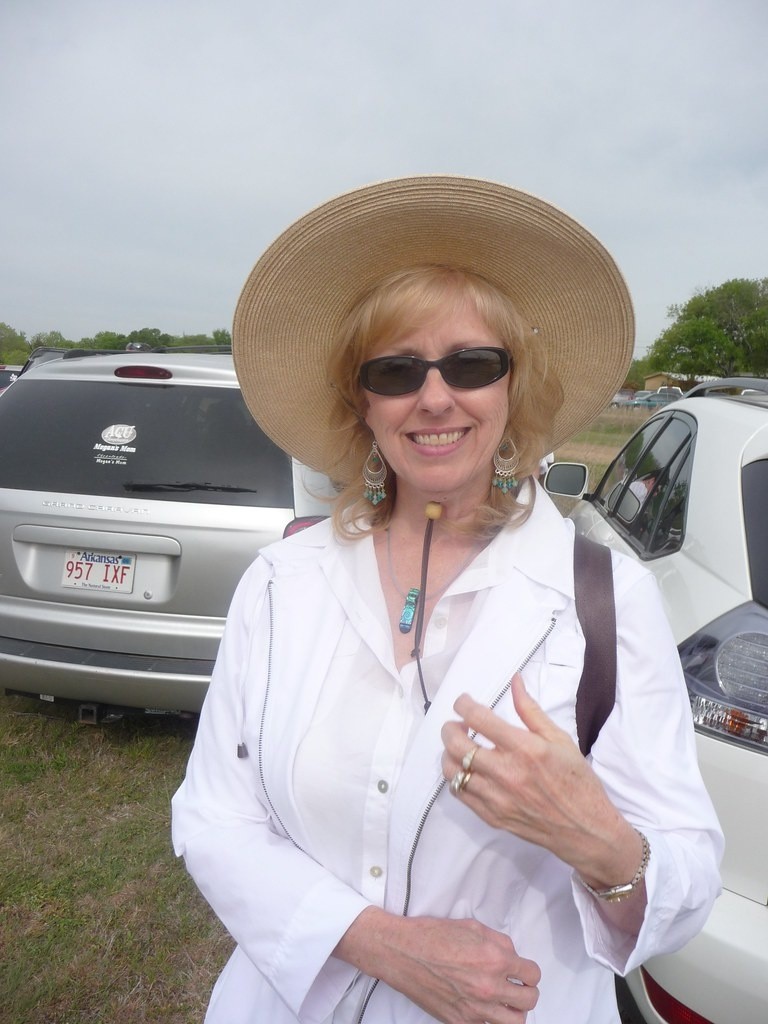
[608,388,652,407]
[621,393,682,408]
[656,386,682,395]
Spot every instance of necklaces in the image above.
[386,503,486,635]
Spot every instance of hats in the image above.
[232,176,635,483]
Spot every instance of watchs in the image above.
[577,826,650,904]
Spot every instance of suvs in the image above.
[541,377,768,1024]
[0,345,553,712]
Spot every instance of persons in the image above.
[171,257,727,1024]
[604,465,671,540]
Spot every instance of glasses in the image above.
[357,345,512,396]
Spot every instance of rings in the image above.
[448,770,471,799]
[461,743,480,774]
